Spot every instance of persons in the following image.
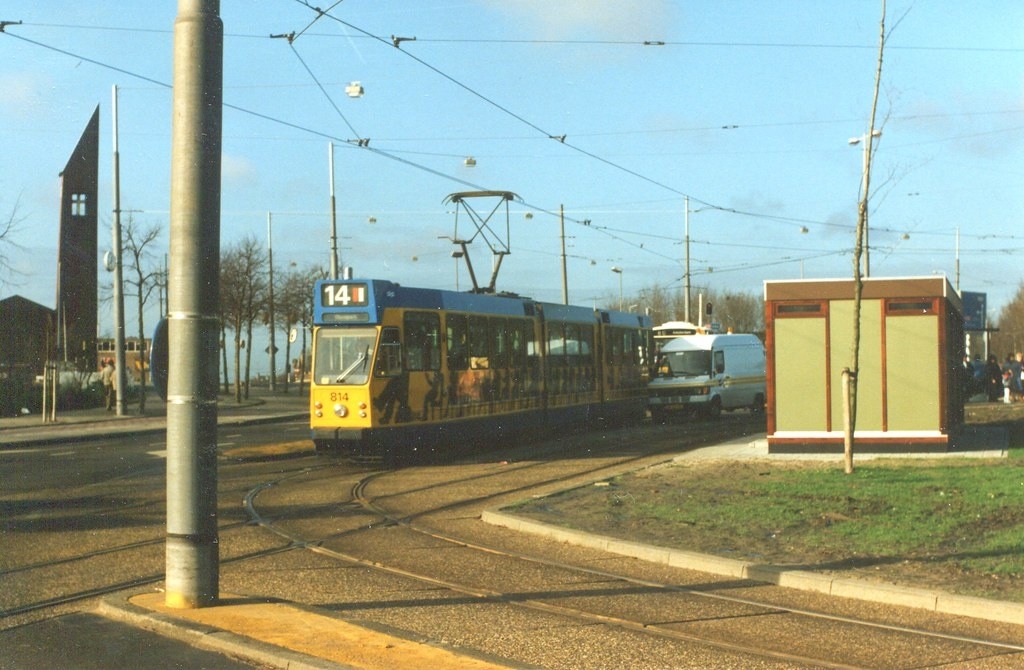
[377,347,397,371]
[962,351,1024,403]
[98,356,117,412]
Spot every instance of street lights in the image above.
[451,251,464,291]
[611,267,622,312]
[849,129,883,278]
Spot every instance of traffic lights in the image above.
[706,303,712,314]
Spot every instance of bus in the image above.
[310,279,655,453]
[653,320,766,373]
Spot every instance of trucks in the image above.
[646,333,768,419]
